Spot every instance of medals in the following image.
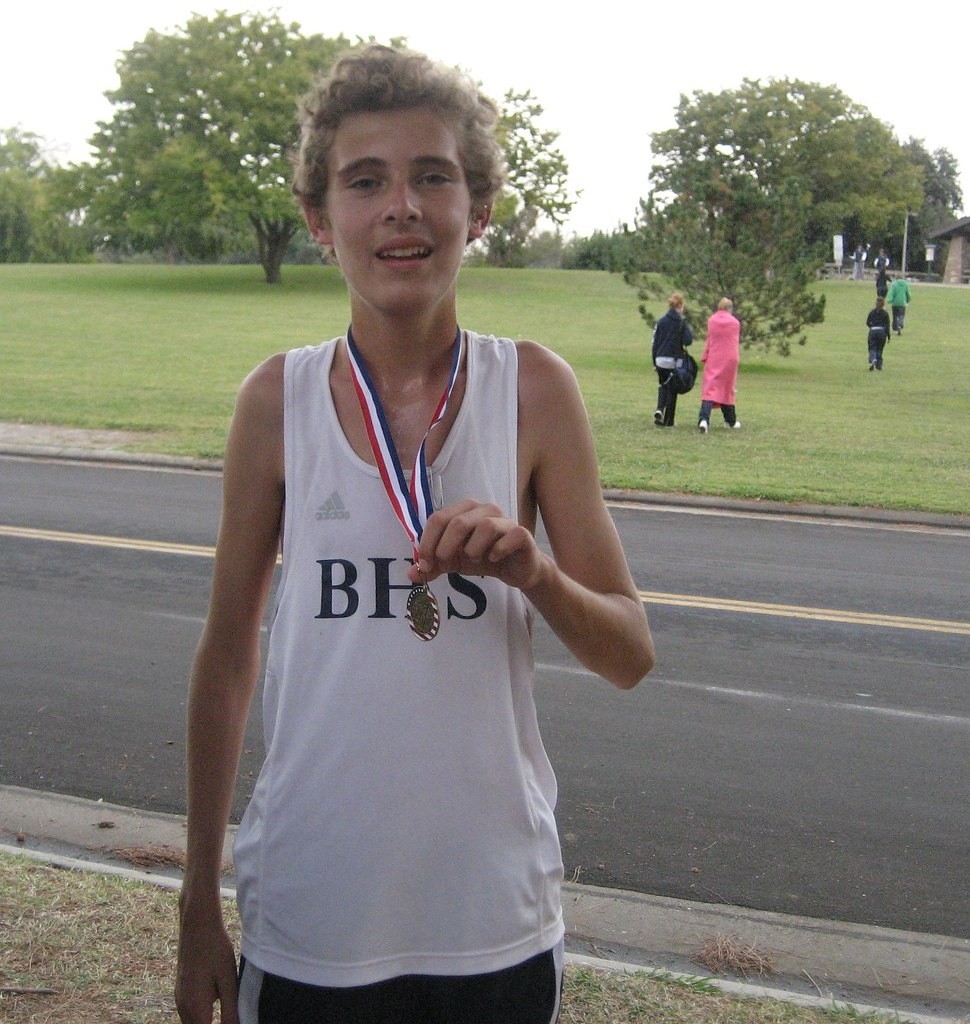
[407,584,440,642]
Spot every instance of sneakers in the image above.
[665,423,677,428]
[724,421,741,428]
[699,420,707,433]
[654,410,663,425]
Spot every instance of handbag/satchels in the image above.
[668,355,697,394]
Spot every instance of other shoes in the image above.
[869,359,877,370]
[897,326,901,334]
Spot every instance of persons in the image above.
[867,297,890,371]
[848,244,867,279]
[887,273,911,335]
[174,44,654,1024]
[697,297,741,432]
[652,295,693,426]
[876,267,892,298]
[874,249,890,270]
